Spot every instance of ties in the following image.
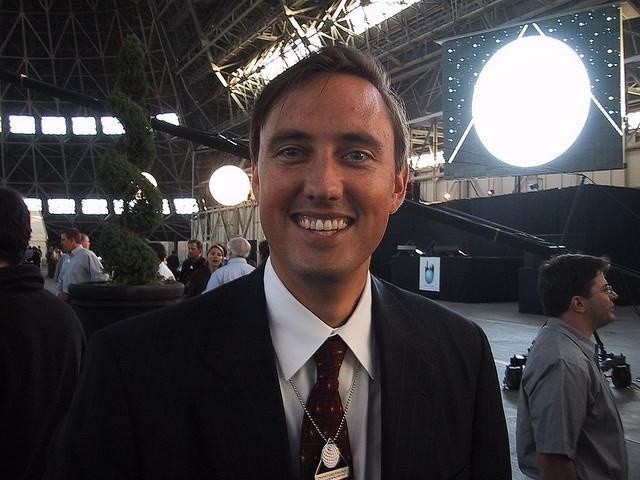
[299,337,353,480]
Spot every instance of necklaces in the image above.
[289,362,362,480]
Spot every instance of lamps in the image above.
[529,182,540,190]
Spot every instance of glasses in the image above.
[590,285,613,295]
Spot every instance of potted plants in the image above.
[65,31,186,326]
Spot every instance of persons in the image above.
[516,253,628,480]
[45,44,512,480]
[23,226,109,301]
[0,188,87,480]
[148,237,269,299]
